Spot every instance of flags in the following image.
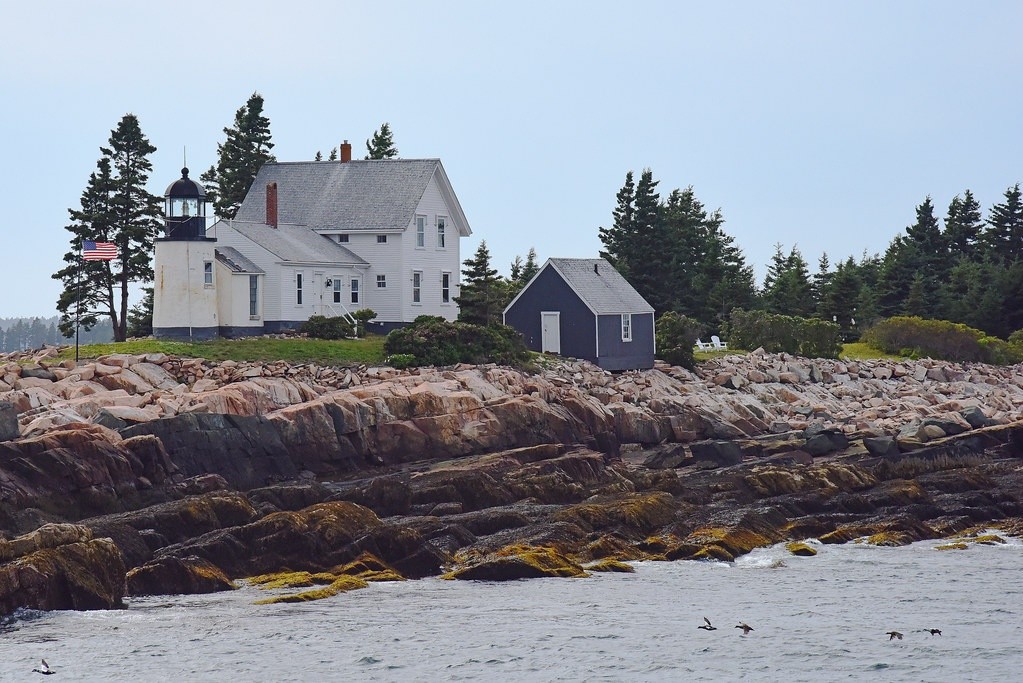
[82,240,118,261]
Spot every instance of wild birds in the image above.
[735,622,756,635]
[923,628,941,635]
[885,631,903,641]
[698,617,717,631]
[31,659,56,676]
